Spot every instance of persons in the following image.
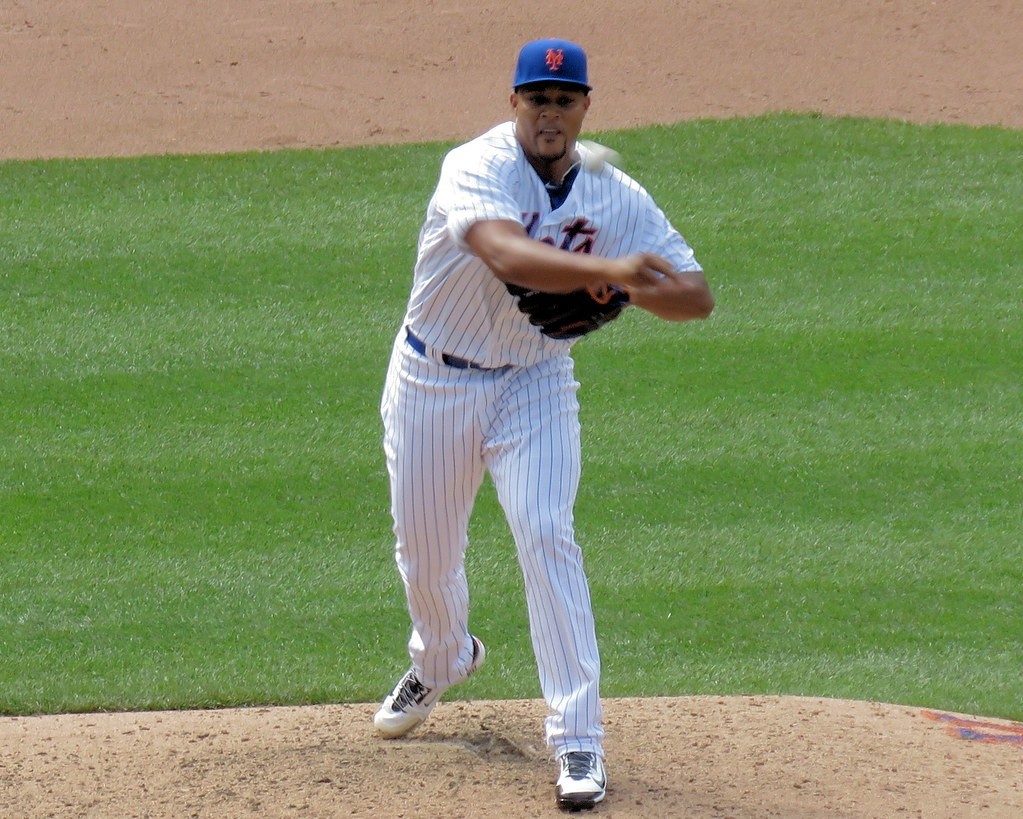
[376,40,715,808]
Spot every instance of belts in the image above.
[407,326,491,371]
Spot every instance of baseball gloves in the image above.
[505,281,633,342]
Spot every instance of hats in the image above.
[513,39,593,91]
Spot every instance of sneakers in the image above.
[373,636,486,740]
[553,751,607,811]
